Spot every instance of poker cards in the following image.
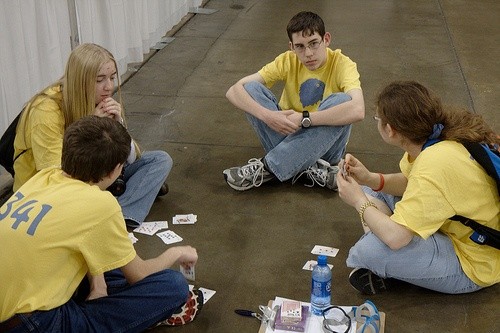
[338,159,349,180]
[311,245,340,258]
[156,230,183,244]
[133,221,168,236]
[302,260,333,272]
[128,232,138,244]
[172,214,197,224]
[199,287,217,304]
[179,262,196,282]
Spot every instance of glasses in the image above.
[290,36,323,53]
[119,160,128,168]
[372,113,382,121]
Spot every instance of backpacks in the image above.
[0,105,30,178]
[420,138,500,249]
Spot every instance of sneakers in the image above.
[292,158,349,192]
[223,156,276,191]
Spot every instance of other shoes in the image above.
[348,267,412,296]
[147,288,204,330]
[157,182,169,196]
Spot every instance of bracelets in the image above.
[360,201,378,227]
[372,172,385,192]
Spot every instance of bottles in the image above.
[310,255,332,316]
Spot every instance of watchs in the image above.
[301,110,312,129]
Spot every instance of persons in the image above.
[12,42,174,228]
[222,10,366,192]
[0,114,199,333]
[336,81,500,295]
